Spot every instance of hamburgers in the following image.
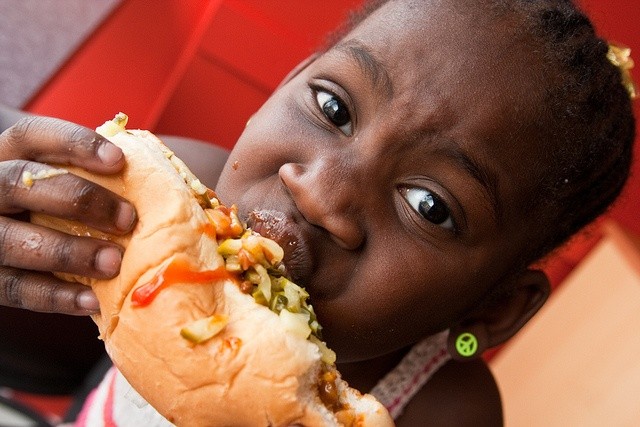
[29,111,397,426]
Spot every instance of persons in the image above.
[1,0,636,427]
